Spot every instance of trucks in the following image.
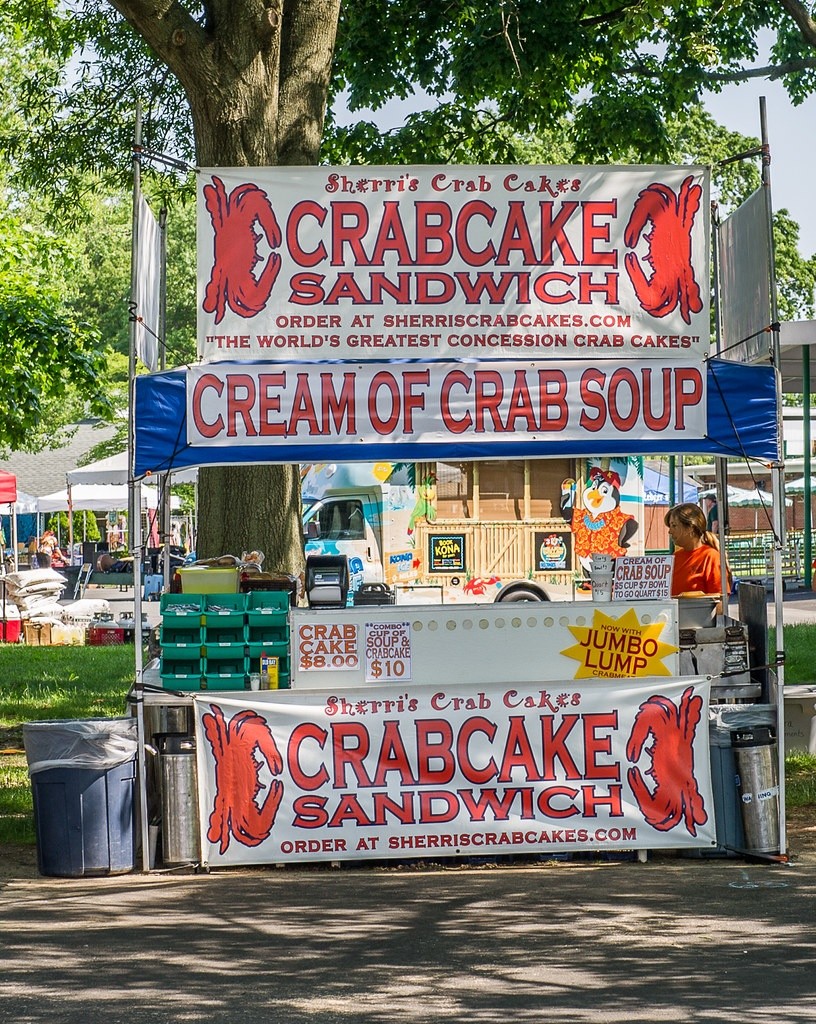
[299,454,645,607]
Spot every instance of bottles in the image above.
[260,672,270,690]
[250,672,261,691]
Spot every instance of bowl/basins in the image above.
[591,552,612,562]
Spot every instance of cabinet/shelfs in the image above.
[158,592,293,690]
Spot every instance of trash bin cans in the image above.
[780,685,816,760]
[730,724,781,864]
[22,717,139,879]
[158,734,202,869]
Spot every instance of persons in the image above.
[702,494,720,534]
[662,502,732,601]
[42,530,63,561]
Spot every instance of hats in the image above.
[702,494,716,500]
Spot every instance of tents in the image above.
[1,469,19,575]
[0,488,42,558]
[133,358,791,873]
[64,446,198,580]
[640,461,700,509]
[35,480,158,576]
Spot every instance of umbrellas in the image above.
[726,486,794,534]
[784,473,816,530]
[697,481,751,499]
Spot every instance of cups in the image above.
[589,560,613,571]
[588,571,615,602]
